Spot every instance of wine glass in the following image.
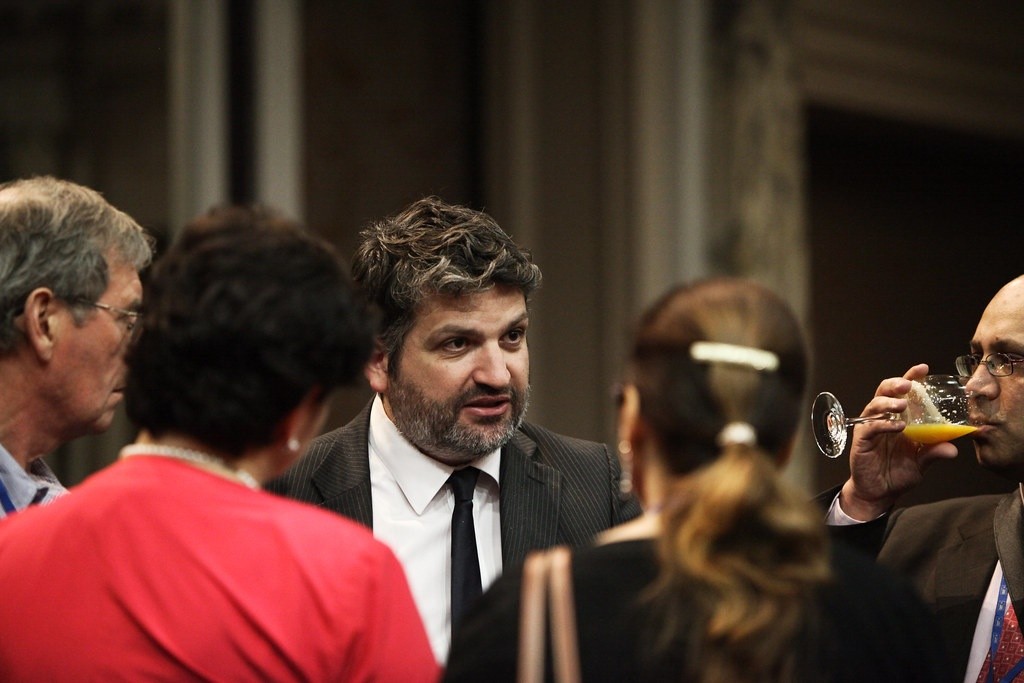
[811,374,993,458]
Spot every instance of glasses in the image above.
[9,293,145,347]
[956,353,1024,377]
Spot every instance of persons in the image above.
[804,272,1024,683]
[260,196,643,683]
[0,178,443,683]
[438,277,955,683]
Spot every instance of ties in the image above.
[976,604,1024,683]
[444,465,483,647]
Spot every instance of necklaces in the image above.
[119,443,259,490]
